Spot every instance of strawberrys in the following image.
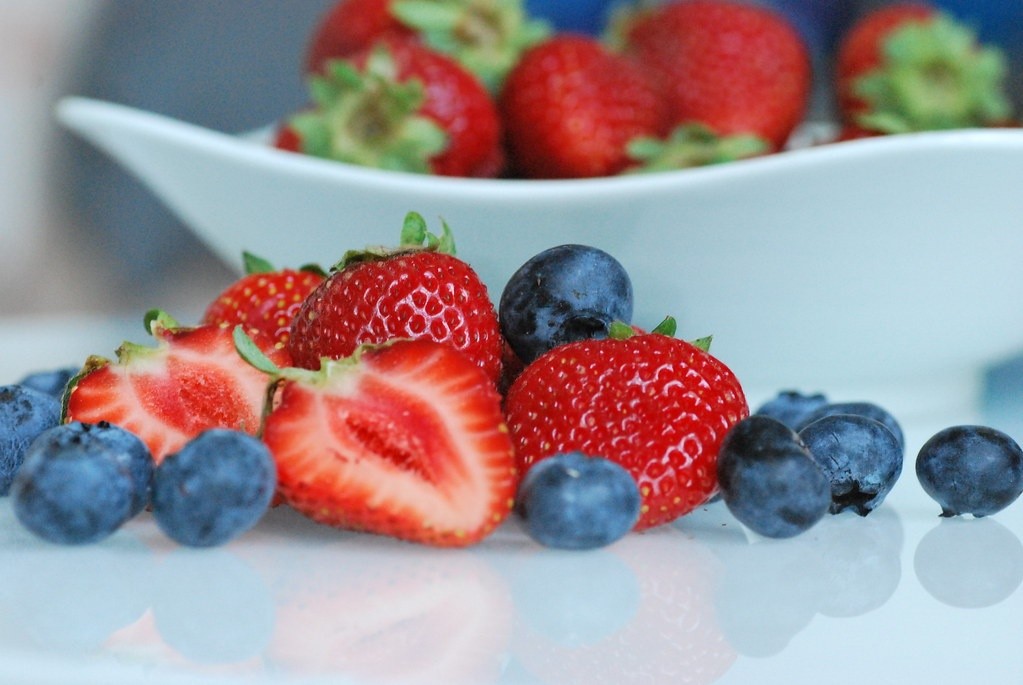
[60,210,750,549]
[270,0,1023,181]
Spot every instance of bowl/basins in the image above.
[48,94,1022,408]
